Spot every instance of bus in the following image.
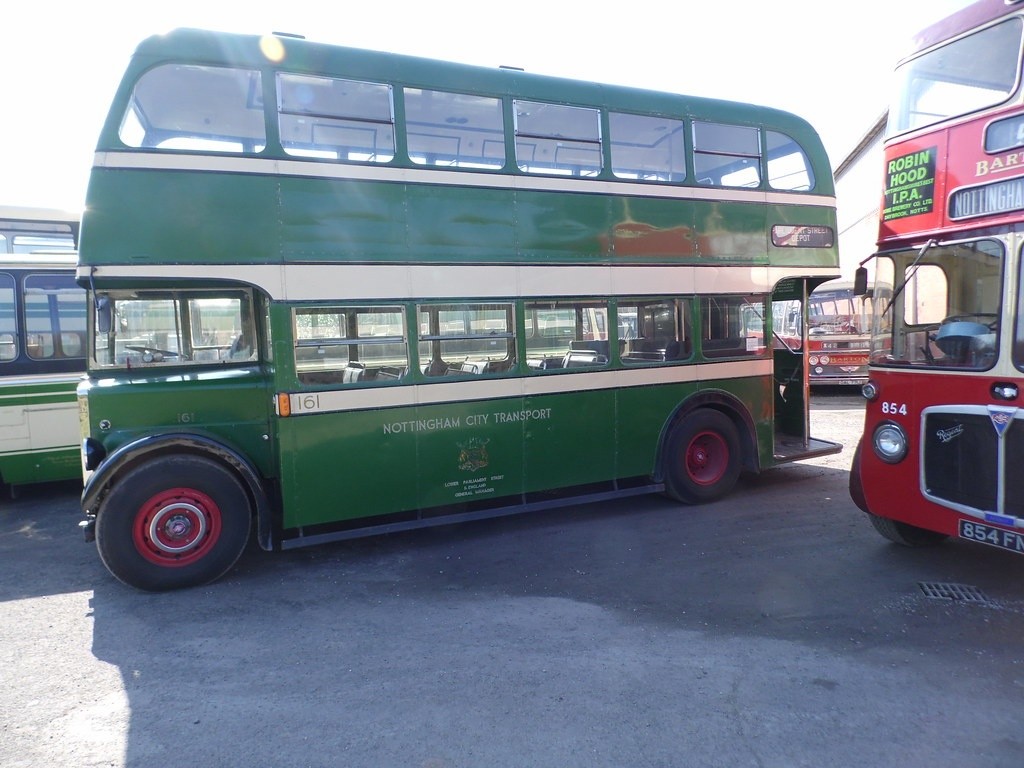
[0,213,84,486]
[848,0,1024,556]
[77,26,844,594]
[742,280,894,385]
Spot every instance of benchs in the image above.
[403,359,431,376]
[461,355,490,374]
[341,361,366,383]
[444,361,478,376]
[560,353,608,368]
[620,351,665,363]
[373,365,403,382]
[507,354,547,372]
